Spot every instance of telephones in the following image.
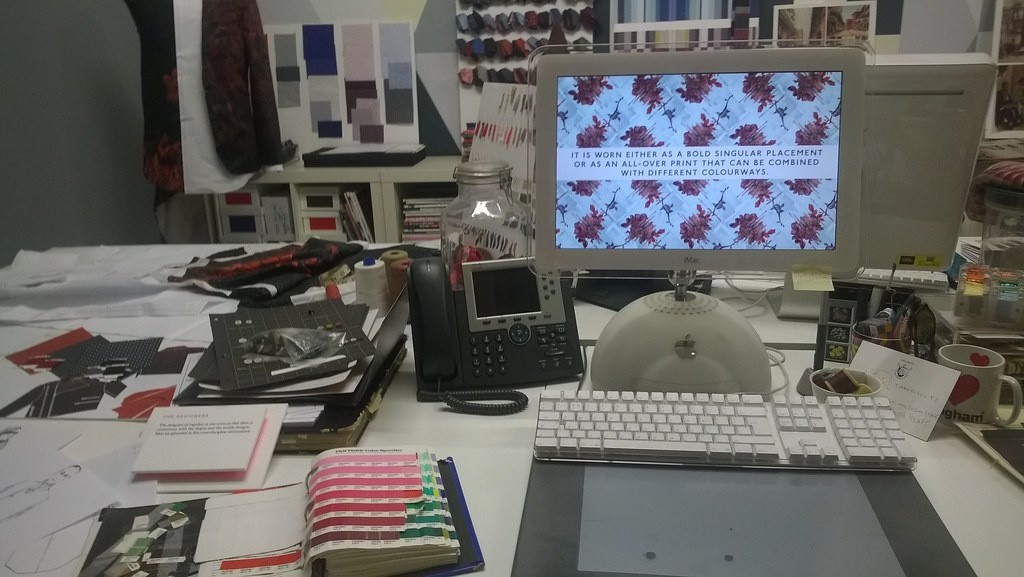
[407,252,586,405]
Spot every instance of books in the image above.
[127,277,419,488]
[192,447,486,577]
[329,183,375,243]
[399,195,456,241]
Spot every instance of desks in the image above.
[0,235,1024,577]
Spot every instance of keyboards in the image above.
[534,389,918,472]
[857,269,949,288]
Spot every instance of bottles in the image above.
[439,160,534,284]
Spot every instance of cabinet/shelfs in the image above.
[218,154,463,246]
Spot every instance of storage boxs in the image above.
[220,184,260,209]
[220,208,266,235]
[300,184,346,210]
[301,211,344,234]
[260,195,293,236]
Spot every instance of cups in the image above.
[810,368,883,405]
[938,344,1022,428]
[849,324,912,369]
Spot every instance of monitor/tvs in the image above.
[529,39,876,400]
[766,53,997,317]
